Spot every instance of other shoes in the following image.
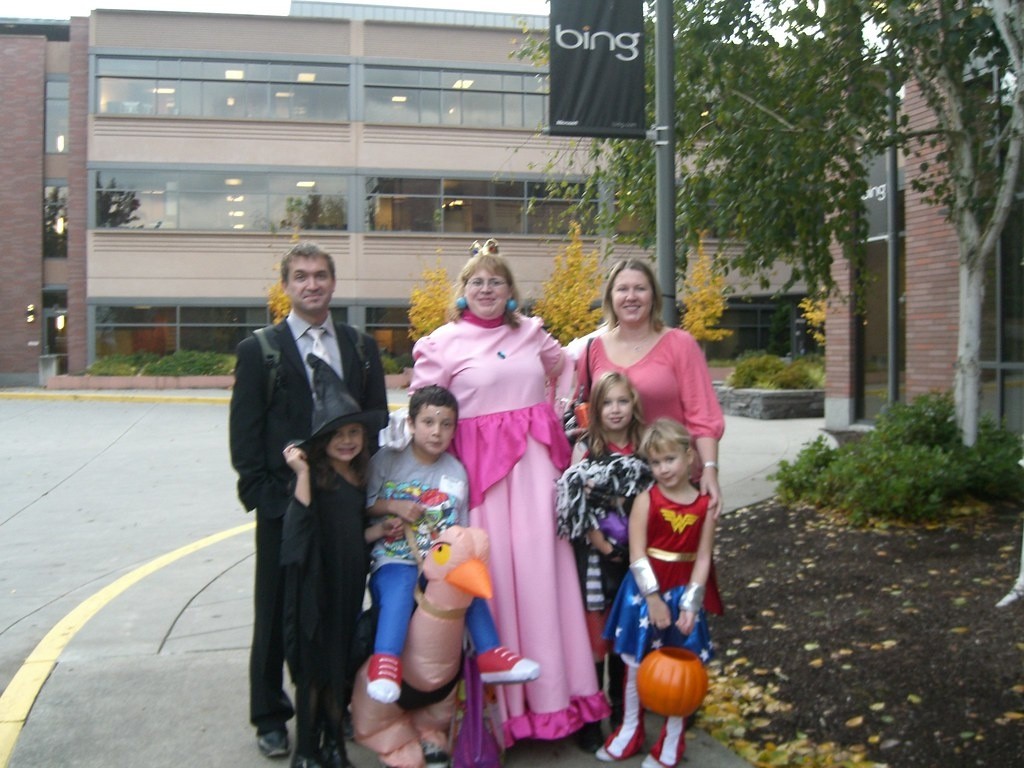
[595,744,615,762]
[641,753,664,768]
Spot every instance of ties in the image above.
[305,328,331,365]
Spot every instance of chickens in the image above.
[350,526,497,767]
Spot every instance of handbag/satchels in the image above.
[563,336,597,445]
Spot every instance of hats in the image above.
[282,352,383,452]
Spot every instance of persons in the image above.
[229,243,389,758]
[555,258,724,768]
[282,352,406,768]
[409,238,611,753]
[366,384,541,704]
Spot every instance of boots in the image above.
[572,660,608,752]
[291,682,328,768]
[605,654,625,739]
[324,692,359,768]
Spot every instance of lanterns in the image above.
[637,648,708,717]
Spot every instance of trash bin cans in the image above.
[39,353,59,386]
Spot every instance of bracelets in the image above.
[702,461,719,471]
[629,556,660,598]
[679,581,705,612]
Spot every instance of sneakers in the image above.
[257,727,291,758]
[367,653,404,704]
[475,646,541,684]
